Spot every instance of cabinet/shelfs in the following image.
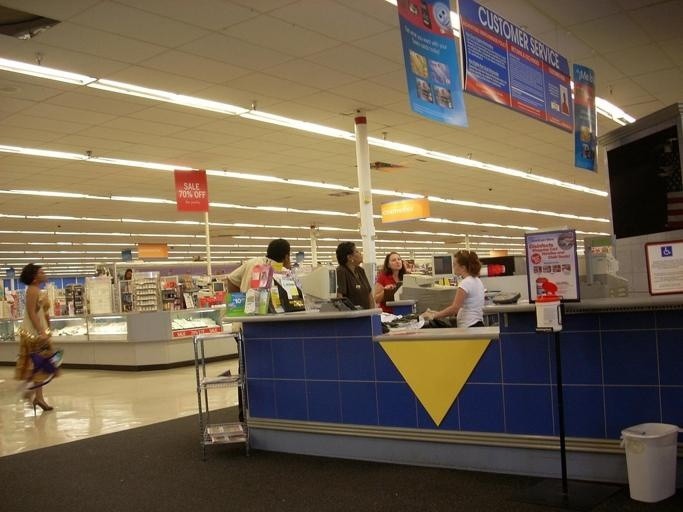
[129,306,237,370]
[1,313,128,366]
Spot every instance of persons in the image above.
[227,239,291,420]
[18,264,53,411]
[122,269,133,279]
[422,251,485,326]
[374,252,407,314]
[336,241,375,309]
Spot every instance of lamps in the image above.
[86,77,250,117]
[0,188,111,200]
[559,213,579,219]
[111,196,177,205]
[352,131,430,156]
[205,167,287,184]
[511,208,537,214]
[288,207,357,217]
[445,198,512,210]
[419,218,479,226]
[208,202,288,213]
[536,210,560,216]
[0,50,99,86]
[479,223,540,231]
[577,216,611,222]
[286,179,397,195]
[85,151,197,172]
[428,150,525,177]
[525,168,608,198]
[239,100,352,139]
[0,144,88,160]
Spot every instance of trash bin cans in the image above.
[620,423,683,503]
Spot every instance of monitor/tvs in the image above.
[213,282,224,292]
[603,125,683,242]
[298,267,337,299]
[433,255,453,278]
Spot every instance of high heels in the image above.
[31,398,54,413]
